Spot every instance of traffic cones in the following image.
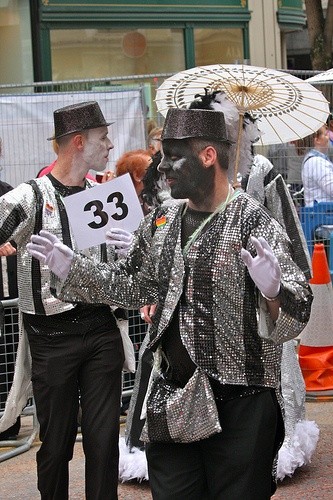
[292,242,333,404]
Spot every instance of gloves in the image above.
[316,225,333,238]
[104,227,135,256]
[242,236,282,299]
[26,229,75,280]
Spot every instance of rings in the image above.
[141,313,144,318]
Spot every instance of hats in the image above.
[46,101,115,140]
[152,108,236,144]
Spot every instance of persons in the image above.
[1,101,135,500]
[116,149,161,220]
[118,91,314,482]
[266,123,333,208]
[26,107,315,500]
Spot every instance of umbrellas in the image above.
[305,68,333,85]
[153,63,332,184]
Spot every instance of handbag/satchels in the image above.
[138,366,223,445]
